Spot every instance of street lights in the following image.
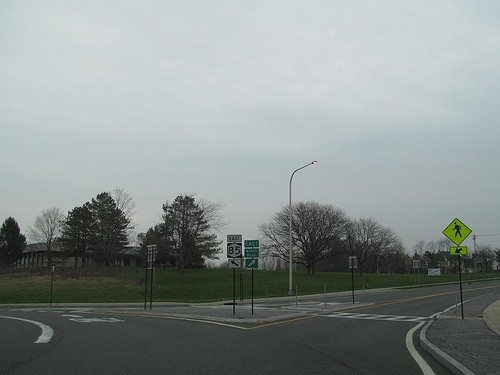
[288,160,317,295]
